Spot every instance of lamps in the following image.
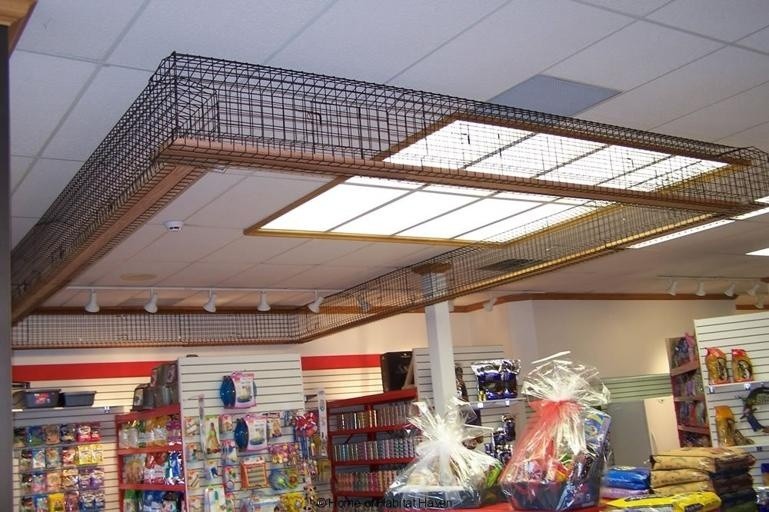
[657,273,769,309]
[65,284,547,315]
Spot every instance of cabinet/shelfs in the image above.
[662,313,769,509]
[115,405,189,512]
[10,404,124,511]
[327,388,422,512]
